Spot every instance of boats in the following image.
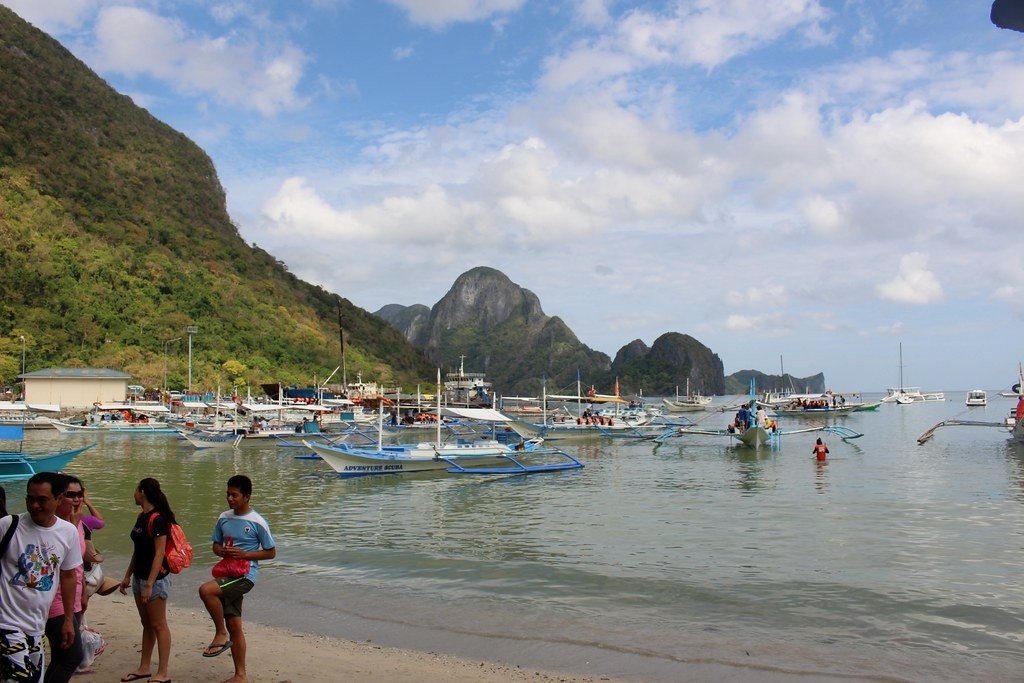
[0,423,98,484]
[755,341,946,418]
[302,365,553,483]
[728,376,774,452]
[965,390,987,406]
[662,376,712,413]
[47,394,677,469]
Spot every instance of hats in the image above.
[96,576,122,596]
[84,540,105,562]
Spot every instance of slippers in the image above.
[146,677,173,682]
[201,640,233,657]
[120,671,154,682]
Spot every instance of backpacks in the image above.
[134,511,194,575]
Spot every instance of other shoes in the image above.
[72,668,97,676]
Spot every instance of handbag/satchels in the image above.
[84,562,104,597]
[78,612,107,668]
[211,535,251,579]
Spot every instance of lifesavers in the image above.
[232,396,243,406]
[377,396,395,406]
[93,402,103,407]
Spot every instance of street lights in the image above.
[20,335,26,401]
[164,337,183,394]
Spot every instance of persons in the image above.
[392,413,437,425]
[120,477,176,683]
[251,416,267,430]
[796,397,828,409]
[727,404,775,433]
[813,437,829,460]
[0,472,83,683]
[45,475,105,683]
[552,414,614,426]
[832,396,843,408]
[199,475,276,683]
[295,415,321,433]
[82,409,130,426]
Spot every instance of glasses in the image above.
[25,495,56,504]
[62,488,86,499]
